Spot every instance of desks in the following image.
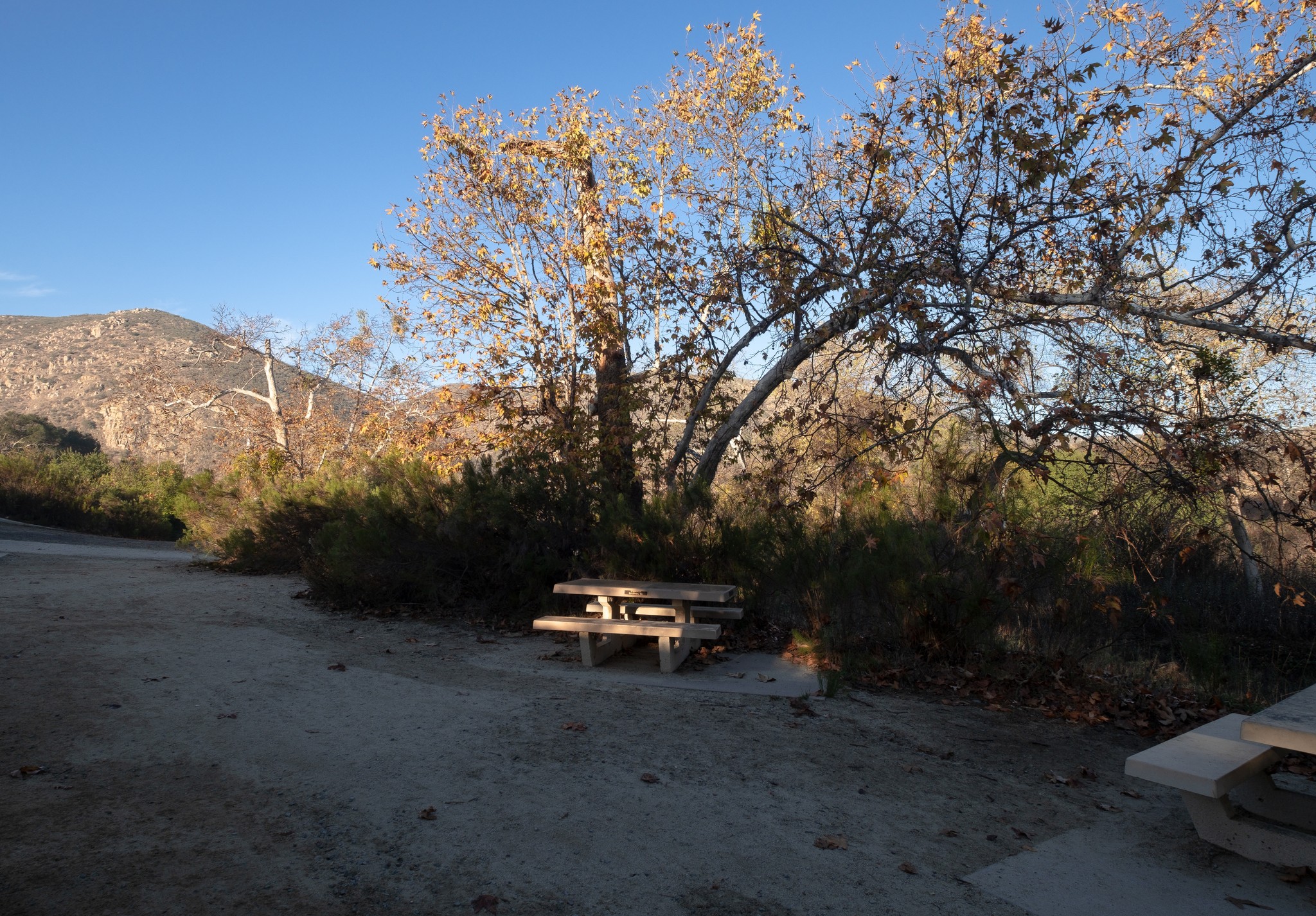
[1199,683,1315,874]
[552,578,739,673]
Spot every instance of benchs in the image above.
[1122,712,1278,862]
[533,616,722,674]
[585,602,744,653]
